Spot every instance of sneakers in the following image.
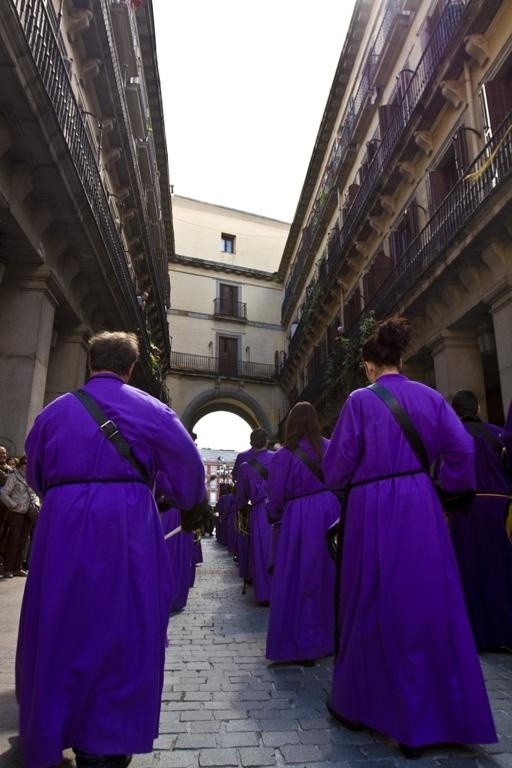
[4,570,28,577]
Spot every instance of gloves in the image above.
[180,497,208,533]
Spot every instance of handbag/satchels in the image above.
[28,504,39,521]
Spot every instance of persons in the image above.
[15,324,210,767]
[1,447,41,580]
[432,388,510,657]
[323,314,498,759]
[154,401,343,670]
